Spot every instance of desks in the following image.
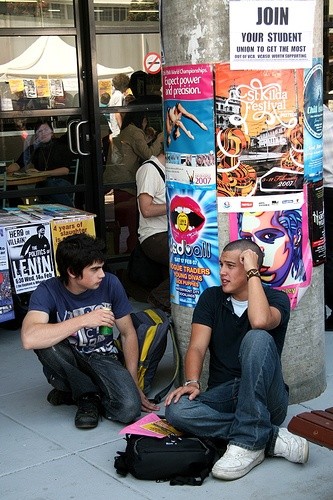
[0,172,47,190]
[0,204,97,324]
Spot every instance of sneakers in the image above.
[274,427,308,465]
[210,445,264,479]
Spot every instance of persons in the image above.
[7,122,72,199]
[7,63,162,142]
[133,144,172,314]
[165,239,310,481]
[105,111,164,183]
[19,225,50,259]
[21,234,160,430]
[166,102,208,147]
[59,116,83,160]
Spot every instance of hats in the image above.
[127,71,146,87]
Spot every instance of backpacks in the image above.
[114,309,179,403]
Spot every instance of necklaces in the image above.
[40,143,54,172]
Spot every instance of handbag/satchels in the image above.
[112,434,226,486]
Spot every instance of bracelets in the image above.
[162,204,166,215]
[246,269,261,280]
[184,379,201,389]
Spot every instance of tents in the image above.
[1,36,134,97]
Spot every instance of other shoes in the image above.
[74,398,98,428]
[46,389,79,405]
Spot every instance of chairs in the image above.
[69,159,79,208]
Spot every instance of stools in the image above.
[127,247,168,291]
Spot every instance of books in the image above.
[0,204,96,323]
